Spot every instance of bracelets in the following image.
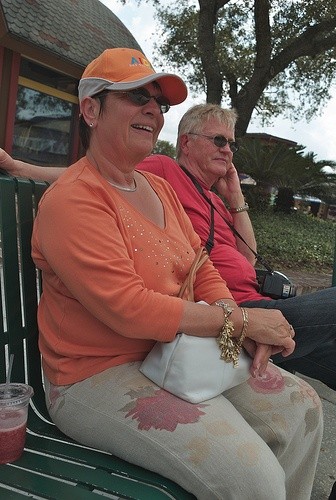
[217,301,248,370]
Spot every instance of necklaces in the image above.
[100,174,139,192]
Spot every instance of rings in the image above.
[289,334,292,338]
[289,325,292,330]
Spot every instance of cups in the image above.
[0,382,34,465]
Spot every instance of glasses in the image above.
[94,86,171,114]
[185,132,240,154]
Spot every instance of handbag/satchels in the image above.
[138,245,253,406]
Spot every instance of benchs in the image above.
[0,174,197,500]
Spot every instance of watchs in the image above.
[230,203,249,213]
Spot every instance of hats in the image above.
[78,48,188,118]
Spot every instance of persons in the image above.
[31,49,323,499]
[0,105,336,389]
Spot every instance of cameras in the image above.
[261,271,296,299]
